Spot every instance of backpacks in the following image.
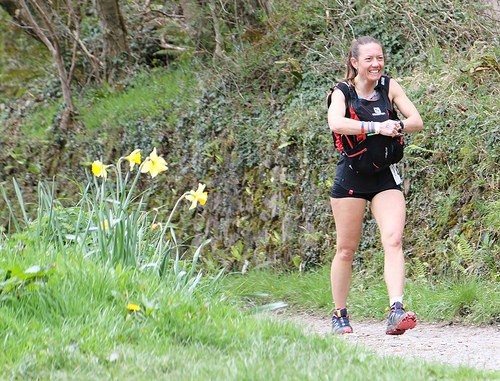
[327,75,404,174]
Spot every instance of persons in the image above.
[327,35,423,335]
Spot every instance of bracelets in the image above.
[367,120,380,134]
[398,120,404,133]
[361,121,365,133]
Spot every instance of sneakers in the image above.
[331,308,353,334]
[385,301,416,336]
